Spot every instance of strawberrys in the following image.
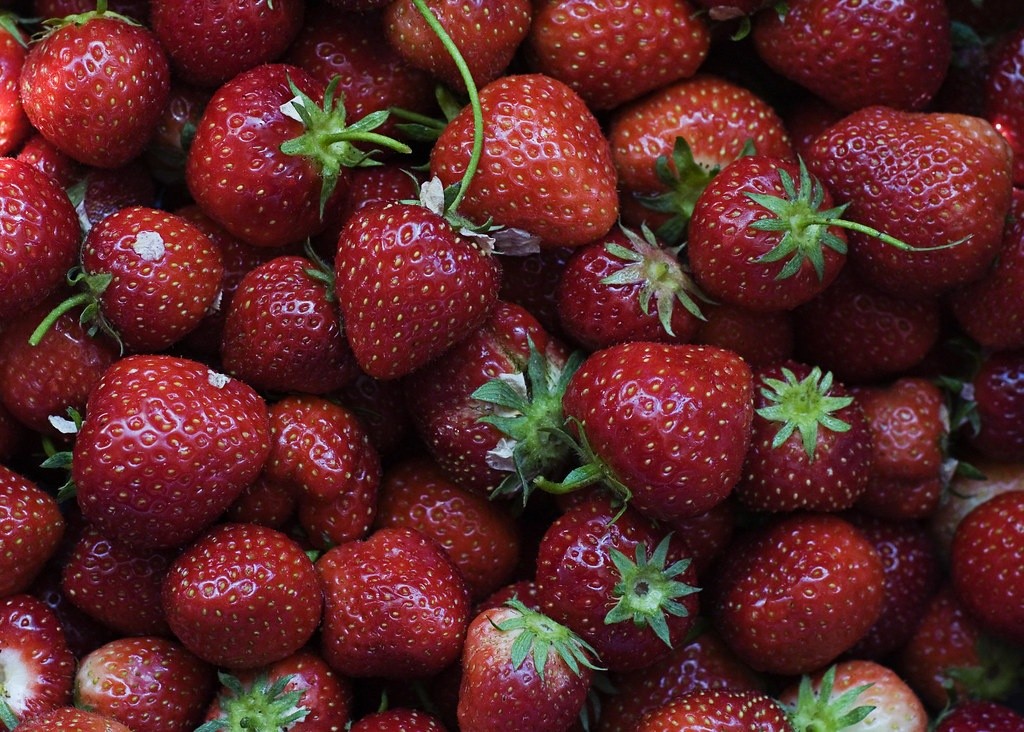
[0,0,1024,732]
